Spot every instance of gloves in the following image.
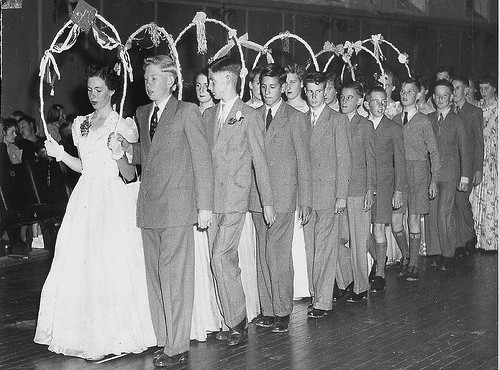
[109,134,124,160]
[44,134,64,162]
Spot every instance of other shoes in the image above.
[395,257,419,280]
[255,316,274,327]
[227,331,248,346]
[430,254,444,267]
[369,275,386,293]
[346,290,367,303]
[215,331,229,340]
[154,348,189,365]
[332,282,353,300]
[306,304,330,318]
[272,315,290,333]
[369,255,388,282]
[440,256,456,270]
[31,235,45,249]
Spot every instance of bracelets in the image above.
[111,150,124,160]
[56,145,64,161]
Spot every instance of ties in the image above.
[439,113,443,122]
[218,104,230,132]
[266,108,272,131]
[457,106,460,115]
[312,112,317,128]
[404,112,408,124]
[150,107,159,141]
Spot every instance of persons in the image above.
[0,55,484,366]
[468,76,498,251]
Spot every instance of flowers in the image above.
[228,111,245,125]
